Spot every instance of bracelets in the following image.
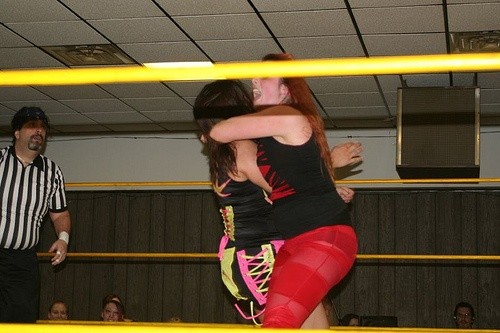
[59,231,69,245]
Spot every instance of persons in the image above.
[192,78,365,329]
[101,294,126,322]
[209,54,358,329]
[340,314,360,327]
[47,300,69,320]
[0,106,72,323]
[453,302,475,329]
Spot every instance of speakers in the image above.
[395,86,481,178]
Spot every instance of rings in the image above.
[56,259,59,263]
[57,251,60,255]
